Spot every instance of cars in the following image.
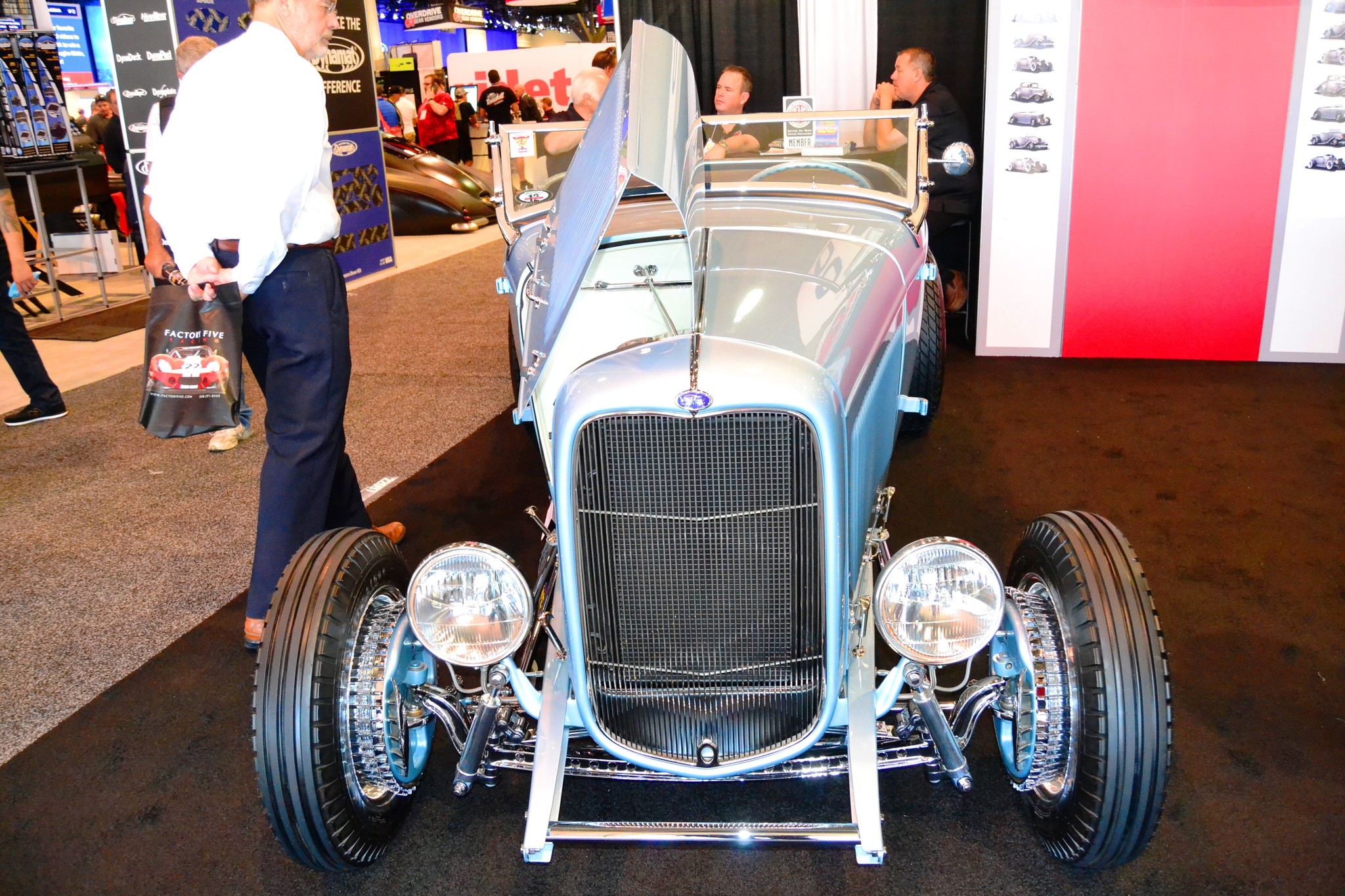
[254,109,1175,877]
[1306,0,1345,171]
[1007,32,1049,176]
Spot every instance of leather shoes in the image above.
[946,271,969,311]
[242,617,265,648]
[372,522,405,542]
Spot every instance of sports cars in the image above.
[377,125,496,233]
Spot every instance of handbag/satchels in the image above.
[136,281,243,438]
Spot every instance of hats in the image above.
[95,94,107,103]
[456,88,470,96]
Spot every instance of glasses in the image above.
[95,95,105,99]
[322,0,338,17]
[423,83,433,86]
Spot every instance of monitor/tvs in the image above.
[450,84,478,113]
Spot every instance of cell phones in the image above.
[431,84,437,93]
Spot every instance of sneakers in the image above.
[5,405,69,427]
[208,421,255,452]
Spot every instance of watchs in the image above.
[718,140,728,151]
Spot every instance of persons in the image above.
[592,51,617,79]
[417,74,464,166]
[513,83,543,123]
[864,45,973,312]
[375,81,418,142]
[453,87,480,166]
[149,0,407,648]
[140,37,255,451]
[540,97,557,121]
[478,70,519,172]
[1,163,69,425]
[604,47,617,63]
[697,64,769,160]
[547,66,610,123]
[69,88,149,277]
